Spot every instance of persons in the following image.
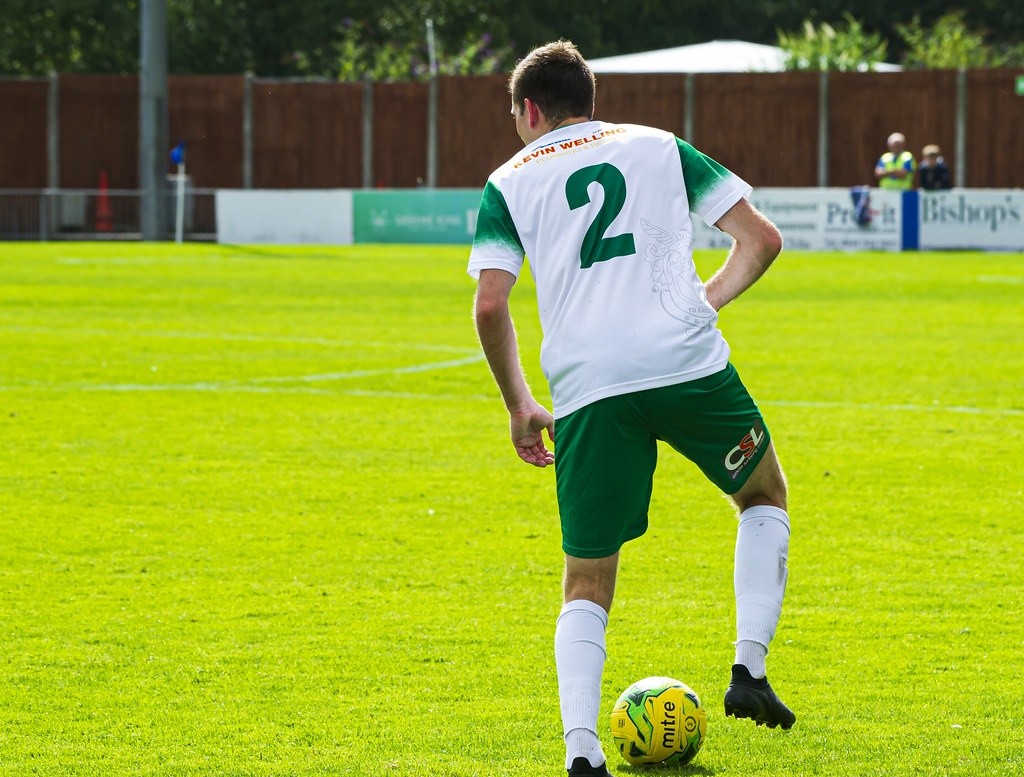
[464,39,798,777]
[918,144,952,189]
[874,132,917,189]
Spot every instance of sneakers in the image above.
[724,664,796,730]
[567,757,613,777]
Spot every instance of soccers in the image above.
[609,675,708,770]
